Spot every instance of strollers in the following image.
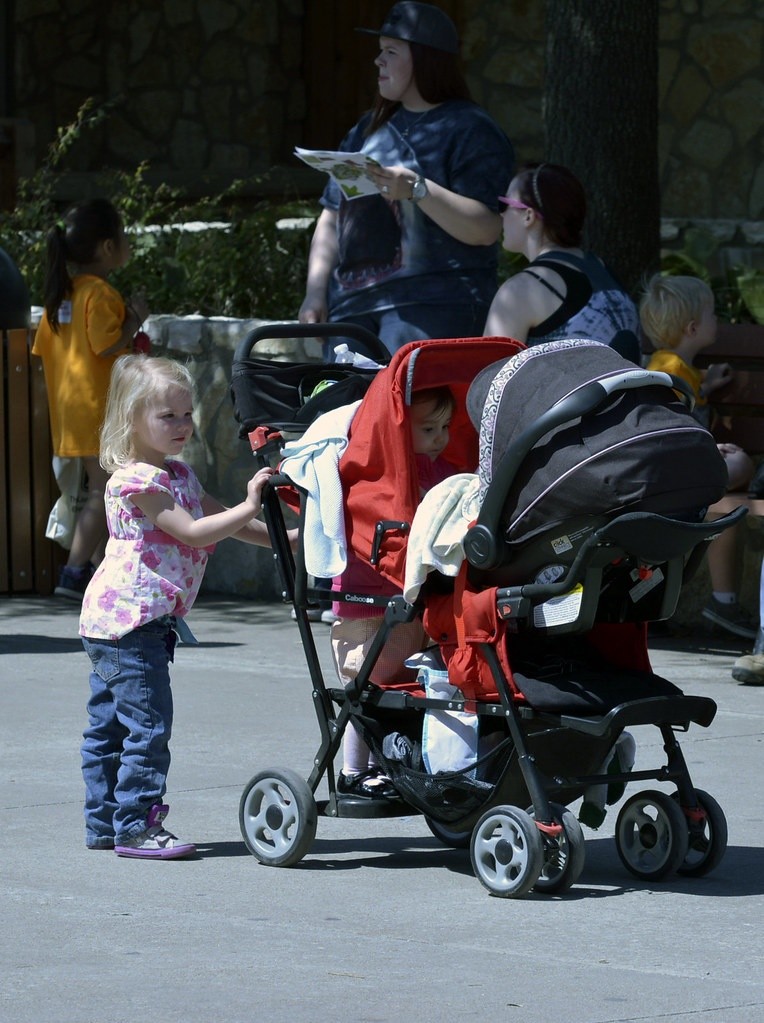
[230,323,750,899]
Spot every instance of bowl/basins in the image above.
[31,305,45,323]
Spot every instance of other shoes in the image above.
[732,654,764,683]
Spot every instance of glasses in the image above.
[497,193,544,219]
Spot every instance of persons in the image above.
[32,201,150,602]
[77,352,307,861]
[330,384,455,805]
[298,0,517,358]
[639,273,756,639]
[483,160,641,368]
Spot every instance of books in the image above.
[295,122,422,199]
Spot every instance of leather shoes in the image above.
[337,769,401,798]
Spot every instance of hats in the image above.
[353,1,458,53]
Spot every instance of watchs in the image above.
[408,172,427,202]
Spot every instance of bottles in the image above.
[334,344,355,363]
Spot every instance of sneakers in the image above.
[54,570,92,602]
[114,805,196,859]
[87,846,113,849]
[702,594,759,638]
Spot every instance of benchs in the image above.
[697,322,764,518]
